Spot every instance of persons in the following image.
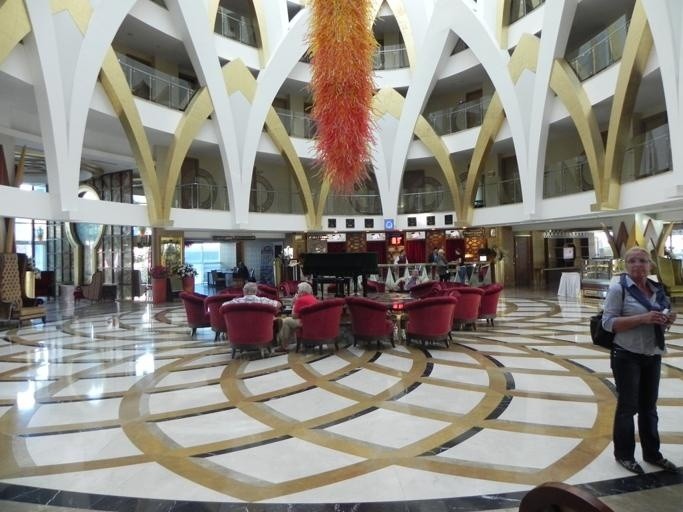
[435,248,448,282]
[273,282,320,352]
[427,247,437,262]
[221,282,282,346]
[601,247,675,474]
[393,249,408,290]
[450,248,463,266]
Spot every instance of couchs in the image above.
[652,256,683,302]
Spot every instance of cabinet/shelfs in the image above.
[546,238,589,269]
[580,258,612,298]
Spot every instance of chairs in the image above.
[0,252,46,324]
[407,280,473,299]
[326,279,387,293]
[219,303,282,357]
[181,290,209,337]
[73,271,102,306]
[435,287,482,331]
[35,271,56,301]
[404,296,459,350]
[278,280,313,296]
[218,281,279,300]
[345,294,397,349]
[204,293,244,342]
[295,296,346,355]
[477,281,503,328]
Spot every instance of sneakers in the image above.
[616,456,644,474]
[643,457,676,471]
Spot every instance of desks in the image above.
[278,305,293,315]
[364,291,412,303]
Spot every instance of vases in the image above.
[151,278,167,303]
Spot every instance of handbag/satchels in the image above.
[590,312,615,349]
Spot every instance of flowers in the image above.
[178,265,197,280]
[149,265,168,278]
